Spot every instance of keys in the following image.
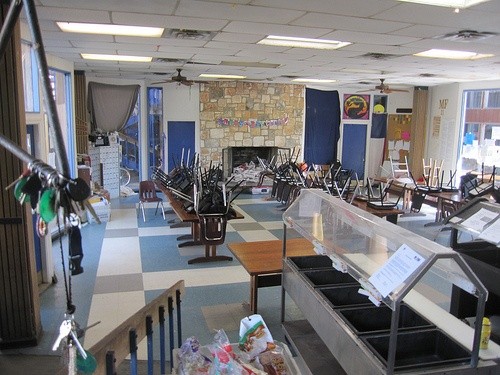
[52,314,100,365]
[5,159,103,237]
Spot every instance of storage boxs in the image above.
[86,205,111,224]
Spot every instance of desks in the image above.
[348,193,404,225]
[153,180,347,314]
[375,177,461,227]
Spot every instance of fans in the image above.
[356,79,410,94]
[149,68,209,86]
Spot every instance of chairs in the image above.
[137,148,245,223]
[256,147,500,210]
